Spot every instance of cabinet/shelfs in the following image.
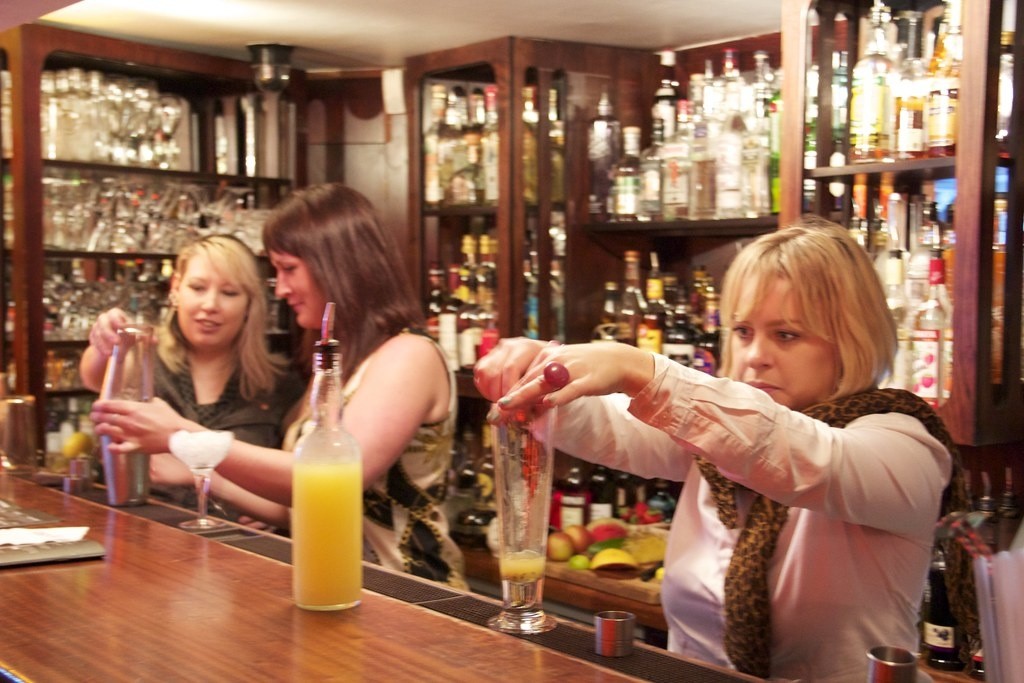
[0,24,310,488]
[405,0,1024,683]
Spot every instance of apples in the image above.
[547,524,590,562]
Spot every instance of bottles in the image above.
[423,0,1024,683]
[290,337,365,613]
[864,644,919,683]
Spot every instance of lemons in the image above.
[568,555,590,570]
[62,431,94,458]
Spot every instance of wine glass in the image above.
[485,403,558,634]
[1,68,289,469]
[166,429,235,530]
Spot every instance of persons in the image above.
[79,235,304,535]
[469,219,956,683]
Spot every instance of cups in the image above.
[0,396,38,477]
[98,324,156,404]
[100,430,149,507]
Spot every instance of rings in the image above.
[544,361,570,391]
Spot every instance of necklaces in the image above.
[89,181,472,592]
